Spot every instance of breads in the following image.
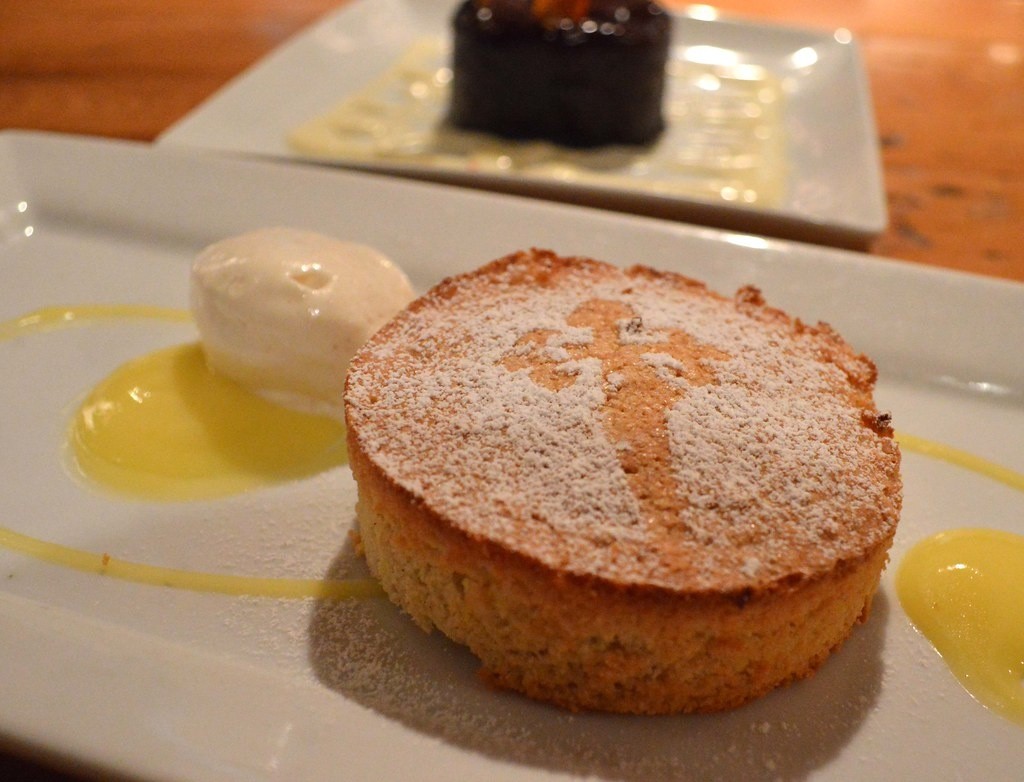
[343,249,902,714]
[450,0,675,148]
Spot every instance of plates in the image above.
[150,0,890,245]
[1,128,1024,781]
